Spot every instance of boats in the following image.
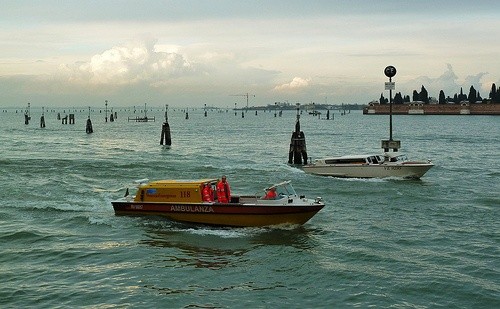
[303,66,435,179]
[111,175,326,227]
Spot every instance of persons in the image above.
[203,182,214,203]
[216,175,231,204]
[267,187,277,198]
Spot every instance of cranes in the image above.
[228,92,256,107]
